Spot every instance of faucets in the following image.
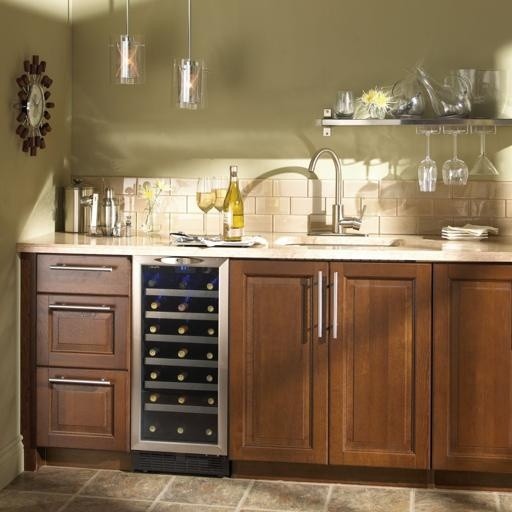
[308,149,367,234]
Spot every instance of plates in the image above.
[440,227,489,241]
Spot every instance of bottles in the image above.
[100,186,118,236]
[126,215,132,236]
[144,269,219,441]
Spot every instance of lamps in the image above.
[106,1,206,111]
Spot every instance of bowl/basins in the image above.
[447,67,509,120]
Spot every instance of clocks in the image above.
[26,82,45,130]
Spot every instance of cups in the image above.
[335,88,355,117]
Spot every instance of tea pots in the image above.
[413,63,473,119]
[386,76,425,119]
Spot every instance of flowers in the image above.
[137,176,171,232]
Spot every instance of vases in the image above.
[143,206,164,231]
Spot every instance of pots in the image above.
[61,175,99,235]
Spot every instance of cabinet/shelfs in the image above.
[17,252,131,457]
[436,260,511,476]
[226,259,432,478]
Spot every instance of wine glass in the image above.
[441,126,469,186]
[195,176,217,240]
[415,125,441,193]
[468,124,500,177]
[212,176,229,242]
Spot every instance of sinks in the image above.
[276,236,442,249]
